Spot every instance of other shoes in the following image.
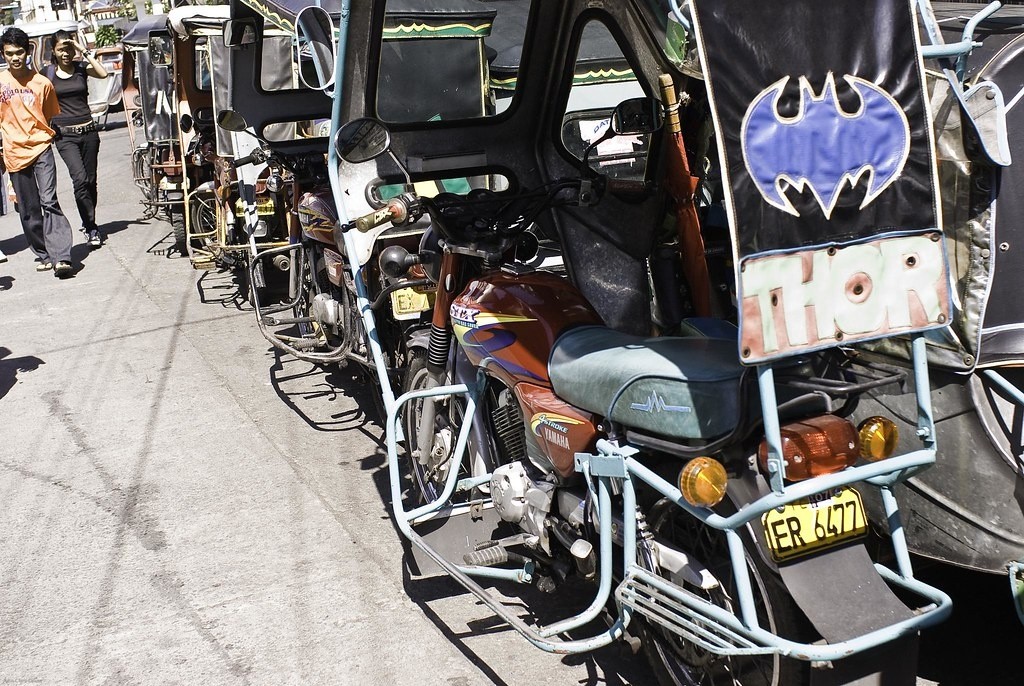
[86,230,101,246]
[0,250,7,263]
[83,227,89,241]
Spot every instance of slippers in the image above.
[37,261,53,271]
[54,262,73,277]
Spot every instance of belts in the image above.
[53,121,97,135]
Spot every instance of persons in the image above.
[38,29,109,248]
[0,26,74,278]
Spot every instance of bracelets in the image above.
[83,51,90,57]
[80,48,86,53]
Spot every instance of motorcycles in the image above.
[117,0,1022,686]
